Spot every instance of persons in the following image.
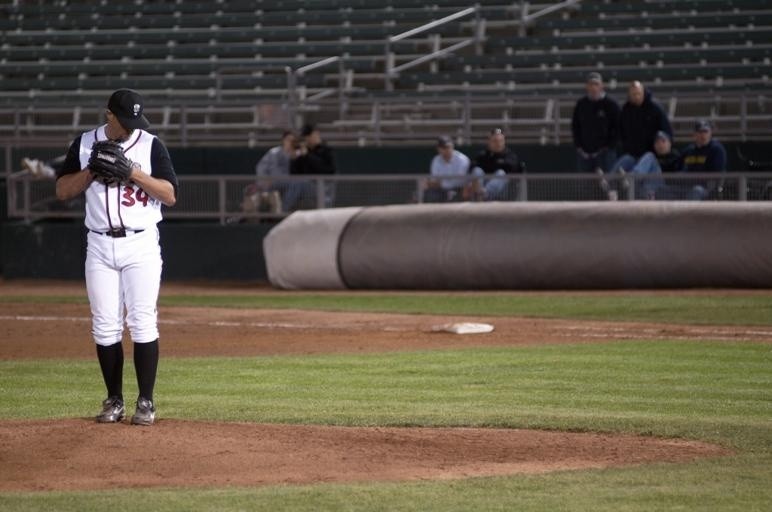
[655,120,727,198]
[412,135,470,204]
[268,123,337,215]
[572,71,622,173]
[460,127,519,200]
[238,130,299,225]
[55,88,180,426]
[621,77,673,158]
[21,140,74,181]
[594,130,681,200]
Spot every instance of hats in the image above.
[299,122,319,137]
[438,134,454,149]
[107,89,151,131]
[694,117,714,134]
[652,129,670,146]
[585,72,602,85]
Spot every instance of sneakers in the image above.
[21,158,60,180]
[130,396,156,426]
[614,165,632,191]
[97,395,125,423]
[594,165,613,195]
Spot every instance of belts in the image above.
[88,228,149,238]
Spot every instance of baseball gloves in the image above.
[88,139,131,181]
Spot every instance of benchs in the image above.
[0,0,771,152]
